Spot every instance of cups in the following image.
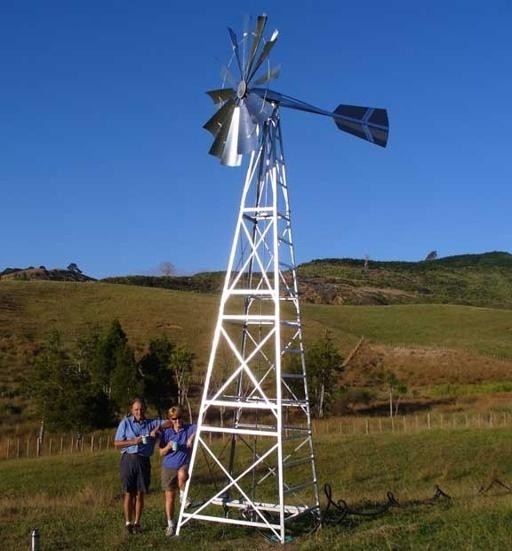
[172,441,178,451]
[141,434,150,445]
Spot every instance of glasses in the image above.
[172,415,182,420]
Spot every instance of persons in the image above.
[157,405,198,536]
[112,396,172,532]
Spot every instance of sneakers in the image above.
[180,497,191,508]
[125,523,143,535]
[165,526,175,537]
